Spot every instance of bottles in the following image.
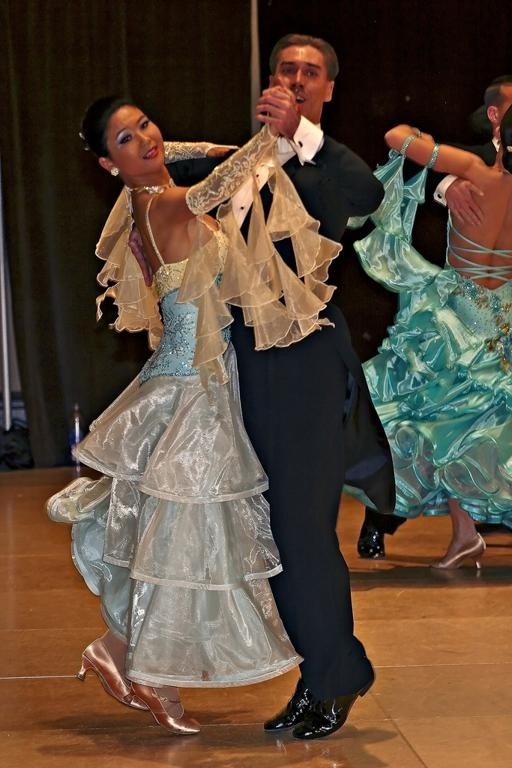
[68,405,85,466]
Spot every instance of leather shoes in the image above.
[358,513,386,560]
[264,670,376,740]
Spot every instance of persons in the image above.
[378,100,511,571]
[221,31,399,740]
[57,91,312,740]
[356,80,512,571]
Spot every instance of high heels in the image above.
[77,637,148,713]
[431,532,486,570]
[121,676,203,736]
